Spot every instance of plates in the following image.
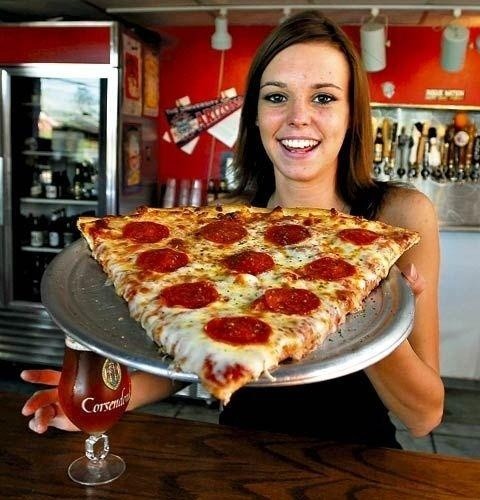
[41,236,414,385]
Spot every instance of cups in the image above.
[164,179,203,209]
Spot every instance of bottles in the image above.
[123,125,142,197]
[206,178,229,206]
[23,157,102,248]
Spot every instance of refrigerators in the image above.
[1,22,160,370]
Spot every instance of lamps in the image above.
[359,9,392,75]
[207,10,237,55]
[439,11,472,71]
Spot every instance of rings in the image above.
[50,402,60,419]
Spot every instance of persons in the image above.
[20,11,445,448]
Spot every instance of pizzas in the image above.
[76,203,421,406]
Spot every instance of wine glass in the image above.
[58,331,129,485]
